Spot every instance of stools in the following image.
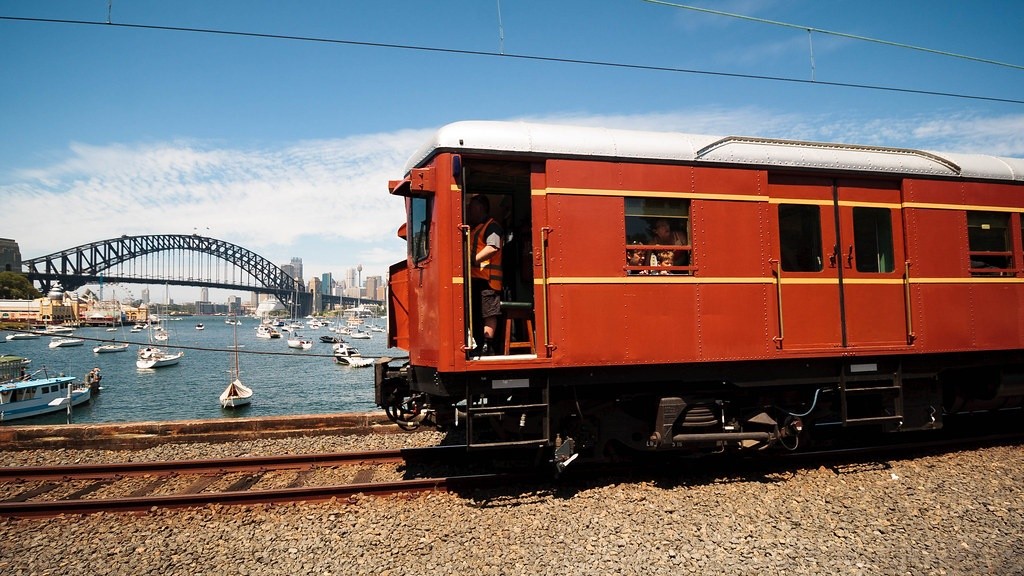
[498,297,535,354]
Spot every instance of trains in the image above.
[374,120,1024,478]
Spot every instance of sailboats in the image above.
[6,294,40,340]
[153,306,168,341]
[347,294,372,339]
[366,305,385,332]
[106,291,118,331]
[319,290,349,343]
[254,300,350,339]
[224,304,242,325]
[48,295,84,348]
[219,310,253,407]
[135,281,183,369]
[195,300,204,330]
[92,290,129,353]
[287,290,314,349]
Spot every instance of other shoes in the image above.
[470,340,495,356]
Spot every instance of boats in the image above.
[45,325,76,333]
[332,341,374,367]
[130,324,148,333]
[172,317,182,321]
[0,353,90,422]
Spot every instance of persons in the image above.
[466,194,504,357]
[625,218,691,274]
